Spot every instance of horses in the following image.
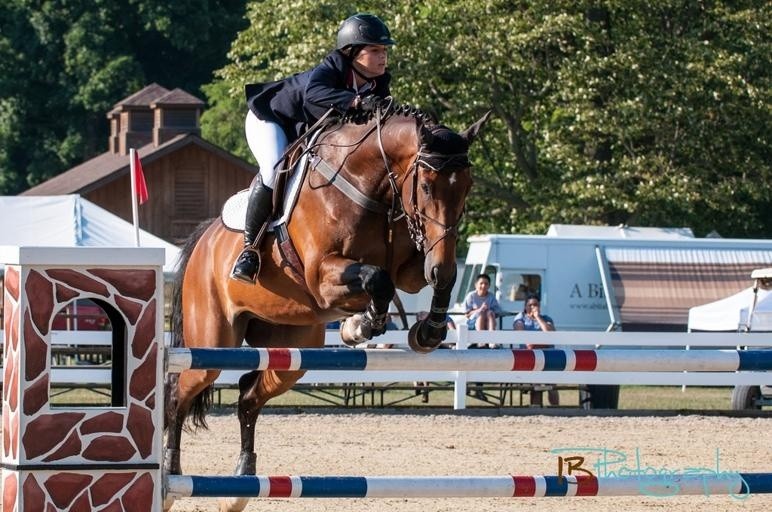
[162,108,493,511]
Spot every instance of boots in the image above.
[233,173,276,281]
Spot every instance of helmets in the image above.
[337,14,395,47]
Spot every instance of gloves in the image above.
[359,93,387,111]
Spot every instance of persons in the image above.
[464,273,502,348]
[414,309,457,403]
[368,322,400,349]
[234,13,394,285]
[511,294,558,405]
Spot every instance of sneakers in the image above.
[476,343,497,349]
